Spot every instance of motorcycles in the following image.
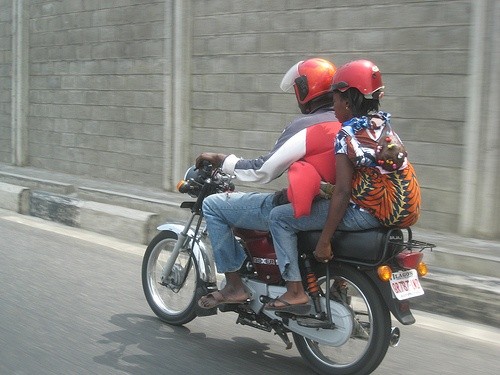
[141,156,438,374]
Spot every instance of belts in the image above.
[352,204,371,214]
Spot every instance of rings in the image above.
[323,259,329,262]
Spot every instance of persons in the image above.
[265,58,420,315]
[194,57,343,308]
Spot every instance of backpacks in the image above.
[341,118,421,228]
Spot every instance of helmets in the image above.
[328,60,385,99]
[293,58,337,115]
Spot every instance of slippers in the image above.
[198,291,249,309]
[265,296,311,316]
[325,292,351,304]
